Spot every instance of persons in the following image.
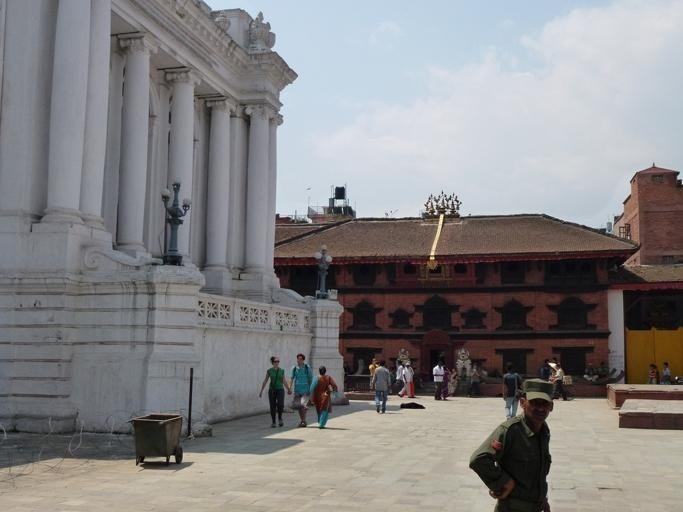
[661,362,672,385]
[468,379,551,512]
[648,363,659,385]
[369,356,610,420]
[289,353,313,427]
[258,355,292,427]
[310,366,339,429]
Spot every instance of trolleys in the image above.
[125,411,185,467]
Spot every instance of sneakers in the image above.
[377,407,380,413]
[279,421,284,426]
[272,423,276,427]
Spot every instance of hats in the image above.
[405,363,410,366]
[521,379,555,405]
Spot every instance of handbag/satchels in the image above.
[514,373,520,401]
[327,383,333,393]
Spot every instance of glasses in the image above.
[274,361,279,363]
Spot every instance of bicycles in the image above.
[548,377,577,401]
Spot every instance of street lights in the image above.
[158,175,192,266]
[312,243,333,298]
